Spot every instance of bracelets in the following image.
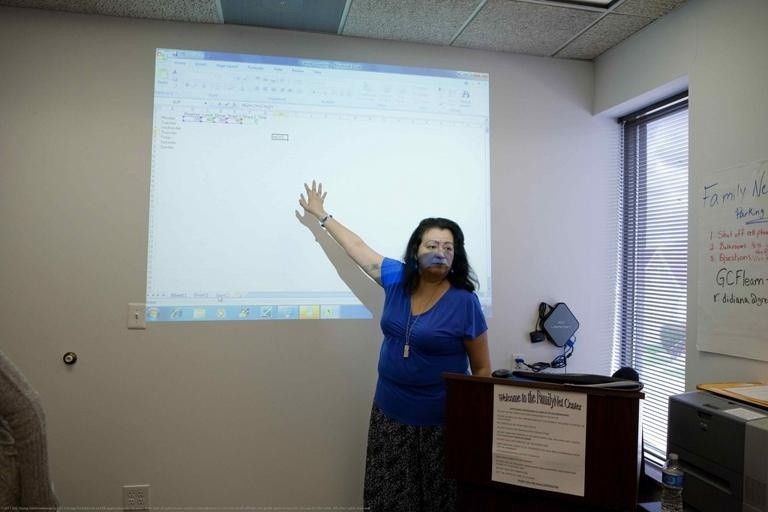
[321,214,332,227]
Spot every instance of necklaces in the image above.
[403,279,445,358]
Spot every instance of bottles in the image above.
[659,453,685,510]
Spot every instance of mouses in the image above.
[492,369,512,377]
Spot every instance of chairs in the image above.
[614,367,647,505]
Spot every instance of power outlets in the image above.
[121,483,151,511]
[512,352,526,370]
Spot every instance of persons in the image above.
[299,181,491,512]
